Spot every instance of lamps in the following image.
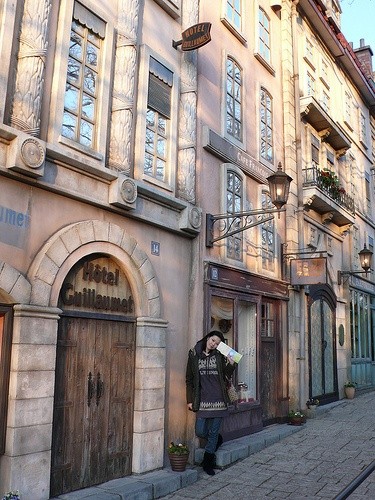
[337,242,375,286]
[205,161,294,248]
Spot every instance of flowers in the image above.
[165,441,191,456]
[286,408,306,418]
[306,396,319,407]
[316,167,344,199]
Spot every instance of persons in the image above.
[185,330,236,475]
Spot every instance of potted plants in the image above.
[344,380,357,398]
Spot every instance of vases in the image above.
[288,417,303,426]
[168,454,189,472]
[308,405,316,411]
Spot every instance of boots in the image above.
[215,433,223,453]
[202,451,216,475]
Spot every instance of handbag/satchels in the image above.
[225,375,238,403]
